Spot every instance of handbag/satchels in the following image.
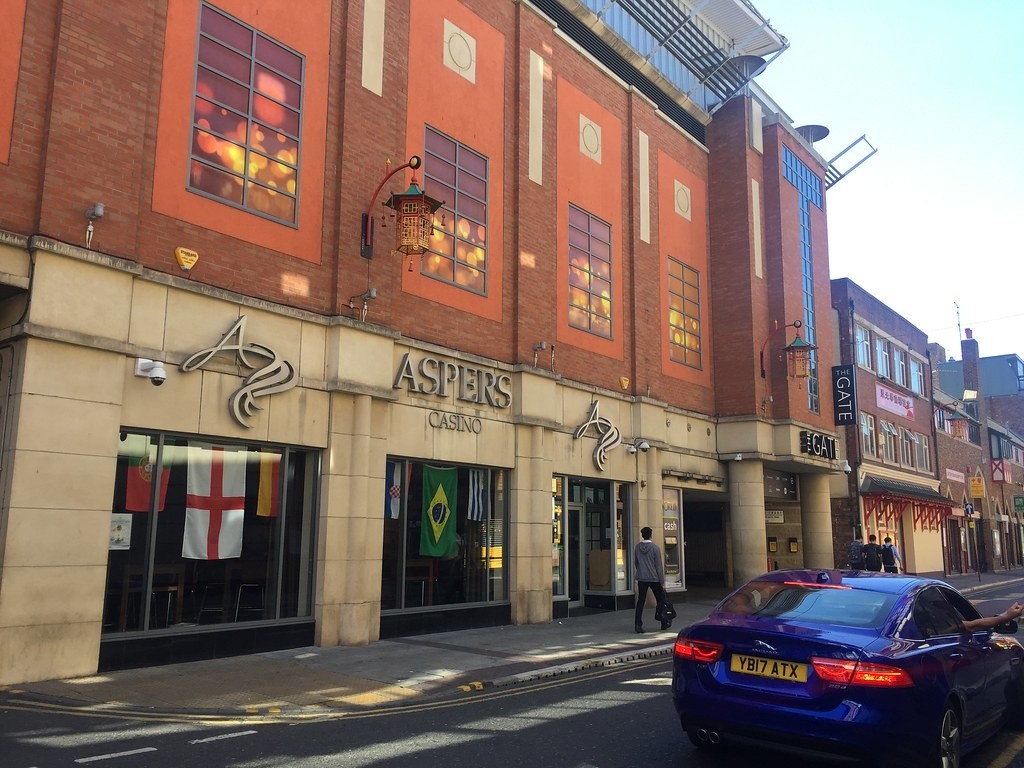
[655,590,677,621]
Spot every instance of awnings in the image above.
[857,473,961,511]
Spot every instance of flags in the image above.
[256,446,296,517]
[467,468,488,521]
[418,463,459,557]
[181,440,249,560]
[384,459,413,518]
[125,435,173,512]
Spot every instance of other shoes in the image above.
[660,621,671,630]
[635,624,645,633]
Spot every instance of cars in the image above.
[672,569,1024,768]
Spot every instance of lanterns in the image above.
[947,412,970,439]
[782,336,819,377]
[382,179,447,260]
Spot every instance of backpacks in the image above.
[865,544,882,571]
[882,544,895,566]
[846,540,863,564]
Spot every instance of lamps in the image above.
[759,320,818,390]
[930,401,969,440]
[360,155,446,272]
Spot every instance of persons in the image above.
[916,606,960,637]
[863,534,884,572]
[881,536,904,575]
[847,534,867,571]
[633,526,671,634]
[960,600,1024,633]
